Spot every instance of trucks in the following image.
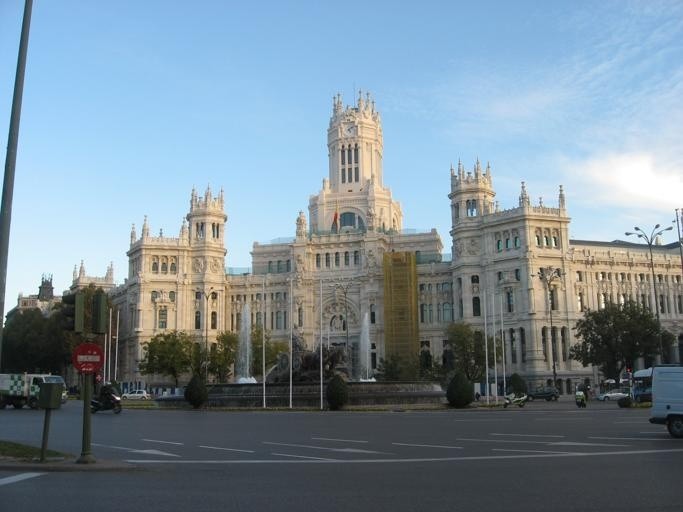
[0,372,67,409]
[649,366,683,437]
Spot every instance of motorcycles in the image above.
[574,385,592,407]
[503,384,527,408]
[90,386,122,414]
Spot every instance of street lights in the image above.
[625,222,673,364]
[335,282,354,353]
[192,286,224,383]
[528,265,566,389]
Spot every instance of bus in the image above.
[628,367,653,404]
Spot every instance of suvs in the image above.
[121,389,151,401]
[525,386,561,402]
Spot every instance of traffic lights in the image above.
[58,293,74,331]
[626,367,632,373]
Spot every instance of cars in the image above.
[597,387,629,400]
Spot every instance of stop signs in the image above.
[72,342,103,376]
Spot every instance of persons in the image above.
[577,380,589,401]
[476,392,480,400]
[96,382,112,404]
